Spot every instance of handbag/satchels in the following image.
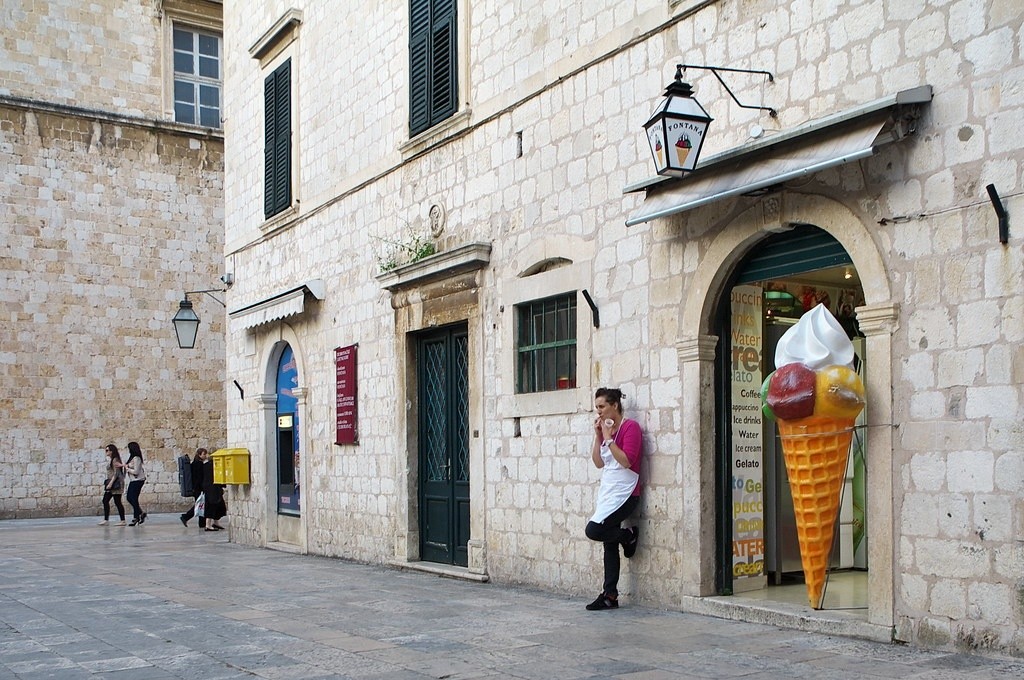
[104,479,121,491]
[194,491,205,517]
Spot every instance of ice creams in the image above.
[762,302,867,610]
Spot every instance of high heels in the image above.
[129,517,140,526]
[138,513,149,524]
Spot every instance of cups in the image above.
[605,419,614,430]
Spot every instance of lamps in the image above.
[173,288,228,349]
[642,64,776,178]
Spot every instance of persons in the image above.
[585,388,643,610]
[98,443,127,527]
[115,441,149,527]
[179,448,207,527]
[202,447,227,532]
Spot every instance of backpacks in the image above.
[178,454,194,497]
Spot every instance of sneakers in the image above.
[620,526,638,558]
[586,590,619,610]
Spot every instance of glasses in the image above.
[106,450,110,452]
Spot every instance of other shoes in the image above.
[180,516,187,527]
[98,521,109,525]
[212,524,225,529]
[114,521,127,526]
[204,527,219,531]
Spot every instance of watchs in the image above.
[607,439,614,446]
